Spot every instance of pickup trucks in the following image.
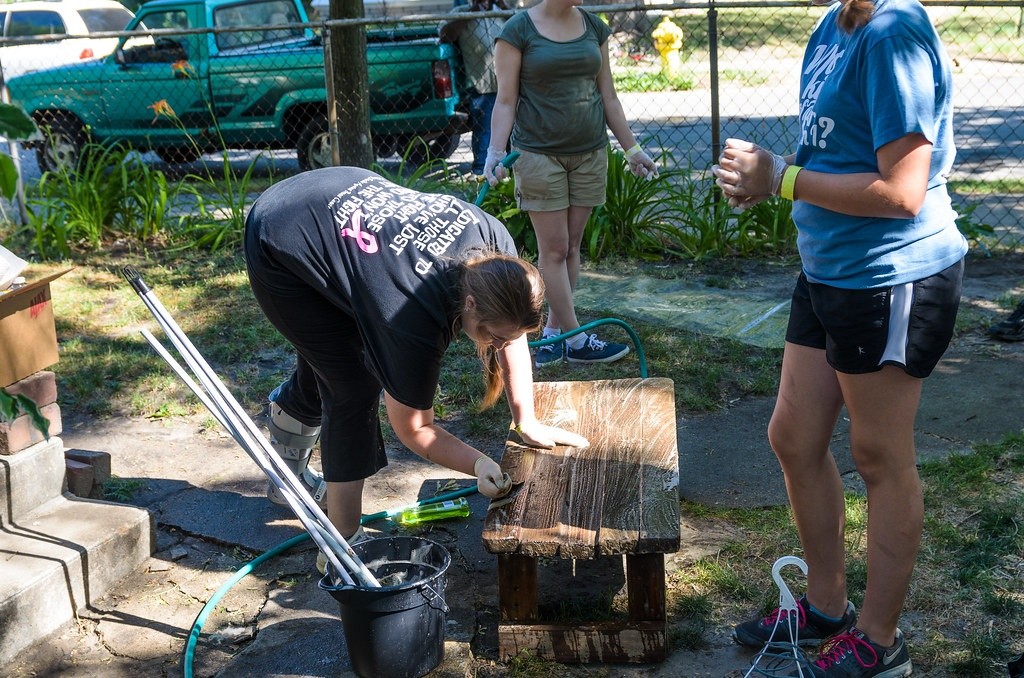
[9,1,495,178]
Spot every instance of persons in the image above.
[438,0,516,181]
[243,166,591,575]
[482,0,658,368]
[711,0,969,678]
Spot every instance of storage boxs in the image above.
[0,265,77,387]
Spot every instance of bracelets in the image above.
[514,422,546,433]
[781,164,805,202]
[625,144,643,157]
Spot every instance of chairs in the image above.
[263,13,291,42]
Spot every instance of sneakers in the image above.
[535,327,563,369]
[315,525,383,575]
[788,625,912,678]
[732,592,855,651]
[566,333,630,363]
[266,469,328,508]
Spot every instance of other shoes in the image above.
[987,303,1024,340]
[467,174,478,182]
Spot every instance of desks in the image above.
[482,377,681,662]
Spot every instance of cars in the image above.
[0,0,155,98]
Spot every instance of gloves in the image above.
[474,455,512,499]
[514,419,590,450]
